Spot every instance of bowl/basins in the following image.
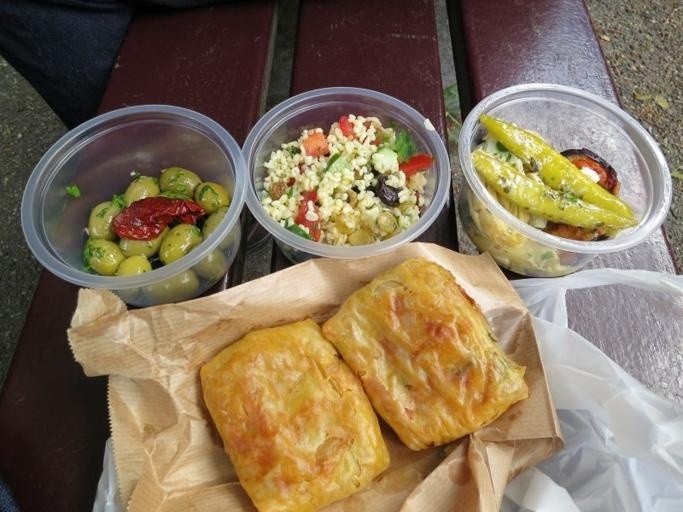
[455,81,672,278]
[20,104,245,309]
[241,85,450,266]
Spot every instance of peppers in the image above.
[479,113,635,220]
[468,149,641,232]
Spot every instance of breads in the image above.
[198,317,391,512]
[320,256,530,454]
